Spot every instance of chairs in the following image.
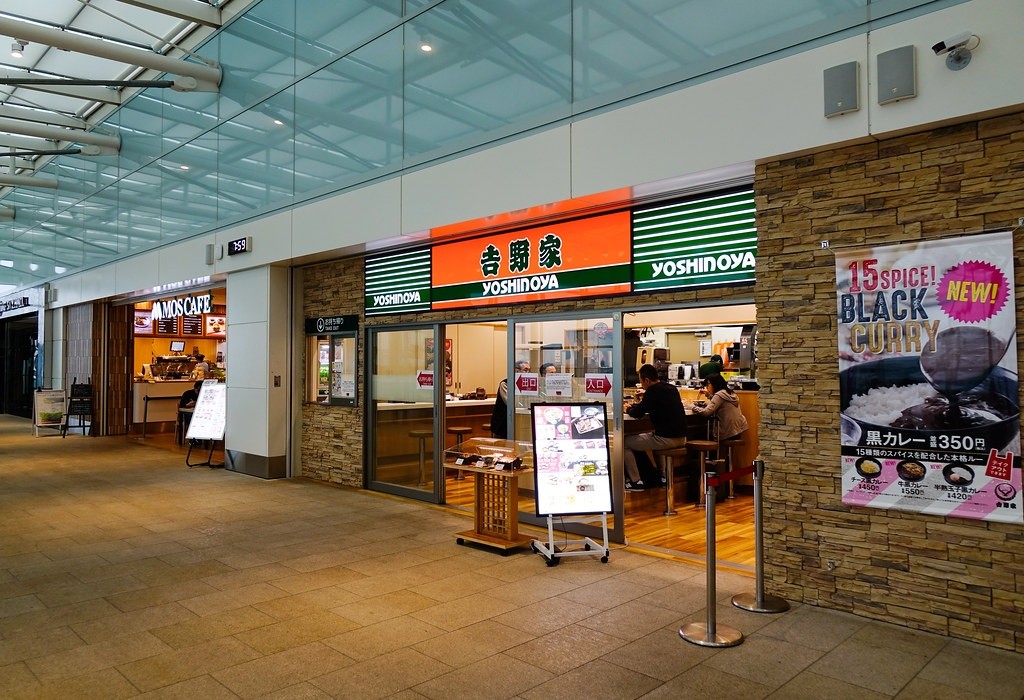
[173,403,182,443]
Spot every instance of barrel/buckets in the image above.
[720,371,739,381]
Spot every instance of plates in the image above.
[134,318,150,327]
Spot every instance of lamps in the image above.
[11,39,29,57]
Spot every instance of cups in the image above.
[693,400,705,407]
[684,366,691,381]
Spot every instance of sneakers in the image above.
[661,478,667,489]
[624,481,645,492]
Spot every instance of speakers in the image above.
[875,44,917,105]
[205,244,214,265]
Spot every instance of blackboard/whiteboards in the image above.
[68,384,93,415]
[185,383,227,440]
[34,389,67,426]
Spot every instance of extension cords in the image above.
[823,61,861,118]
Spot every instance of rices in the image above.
[846,382,940,427]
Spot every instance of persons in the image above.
[490,360,530,439]
[700,354,723,386]
[683,371,750,474]
[177,381,204,448]
[538,363,557,377]
[194,354,209,372]
[622,364,687,492]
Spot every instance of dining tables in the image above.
[179,407,194,445]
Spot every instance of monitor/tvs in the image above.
[169,341,185,352]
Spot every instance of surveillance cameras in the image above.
[932,31,970,55]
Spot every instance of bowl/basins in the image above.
[839,356,1018,455]
[674,380,687,386]
[691,381,700,386]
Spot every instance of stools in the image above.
[410,430,433,486]
[717,440,744,499]
[482,424,497,439]
[448,427,472,480]
[653,447,687,516]
[686,440,719,509]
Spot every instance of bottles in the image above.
[320,350,329,386]
